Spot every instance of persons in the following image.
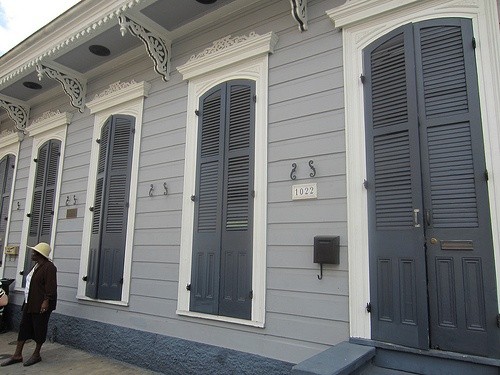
[0,284,8,316]
[0,242,57,367]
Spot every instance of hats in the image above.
[27,243,52,259]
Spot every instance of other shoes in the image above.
[0,357,23,366]
[23,357,41,365]
